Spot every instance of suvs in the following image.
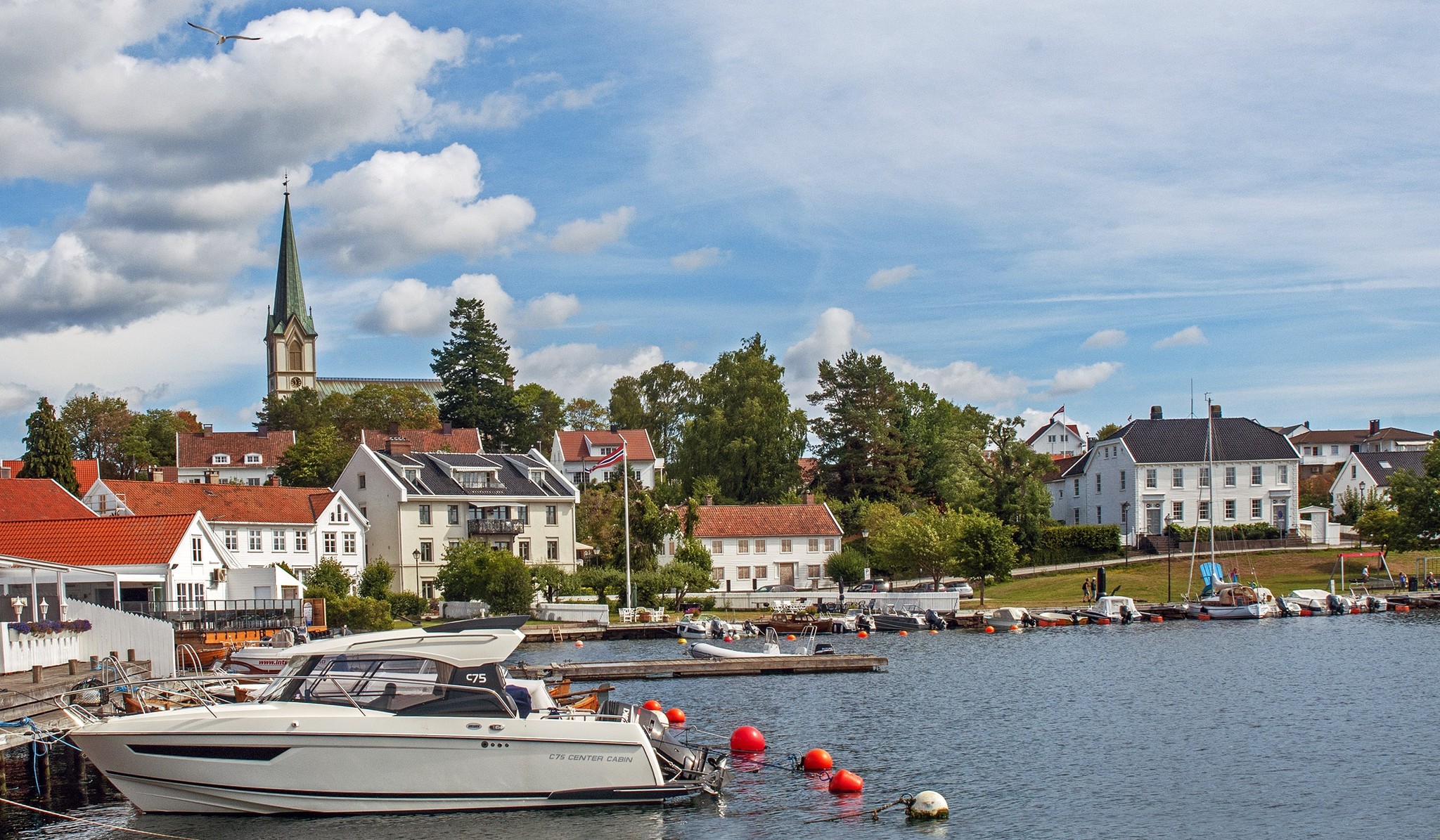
[755,585,797,592]
[847,579,889,593]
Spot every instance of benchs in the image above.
[1422,579,1440,591]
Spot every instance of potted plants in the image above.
[659,614,670,622]
[683,607,701,621]
[425,617,432,622]
[635,608,651,624]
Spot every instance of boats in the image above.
[675,603,1089,640]
[1257,585,1416,618]
[48,616,737,817]
[688,623,835,663]
[1059,596,1144,623]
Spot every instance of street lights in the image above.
[1123,501,1131,566]
[861,528,869,570]
[593,545,601,571]
[1164,513,1174,602]
[1358,481,1366,548]
[412,548,420,596]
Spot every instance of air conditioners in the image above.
[213,569,228,583]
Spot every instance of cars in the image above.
[944,581,974,599]
[909,582,947,593]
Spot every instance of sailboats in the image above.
[1173,397,1270,621]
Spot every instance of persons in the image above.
[1426,572,1440,590]
[1088,577,1097,602]
[1362,565,1370,581]
[1081,578,1091,603]
[1398,571,1407,592]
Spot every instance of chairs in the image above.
[619,606,664,623]
[859,598,877,614]
[771,600,813,617]
[817,600,865,615]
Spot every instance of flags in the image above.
[584,440,627,472]
[1052,405,1064,418]
